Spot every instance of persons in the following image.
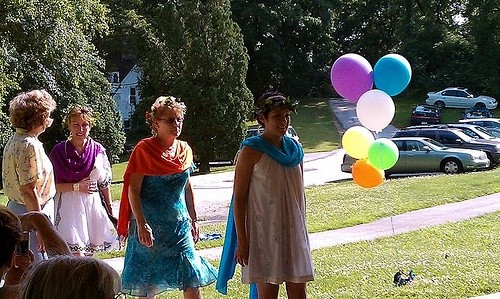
[0,206,74,299]
[215,96,315,299]
[331,52,412,189]
[49,107,117,257]
[2,89,56,265]
[19,256,121,299]
[117,96,218,299]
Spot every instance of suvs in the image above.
[393,118,500,168]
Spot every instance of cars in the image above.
[410,105,440,125]
[341,137,490,177]
[462,107,493,118]
[426,87,498,109]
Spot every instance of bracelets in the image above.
[72,183,80,192]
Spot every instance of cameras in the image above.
[14,233,31,257]
